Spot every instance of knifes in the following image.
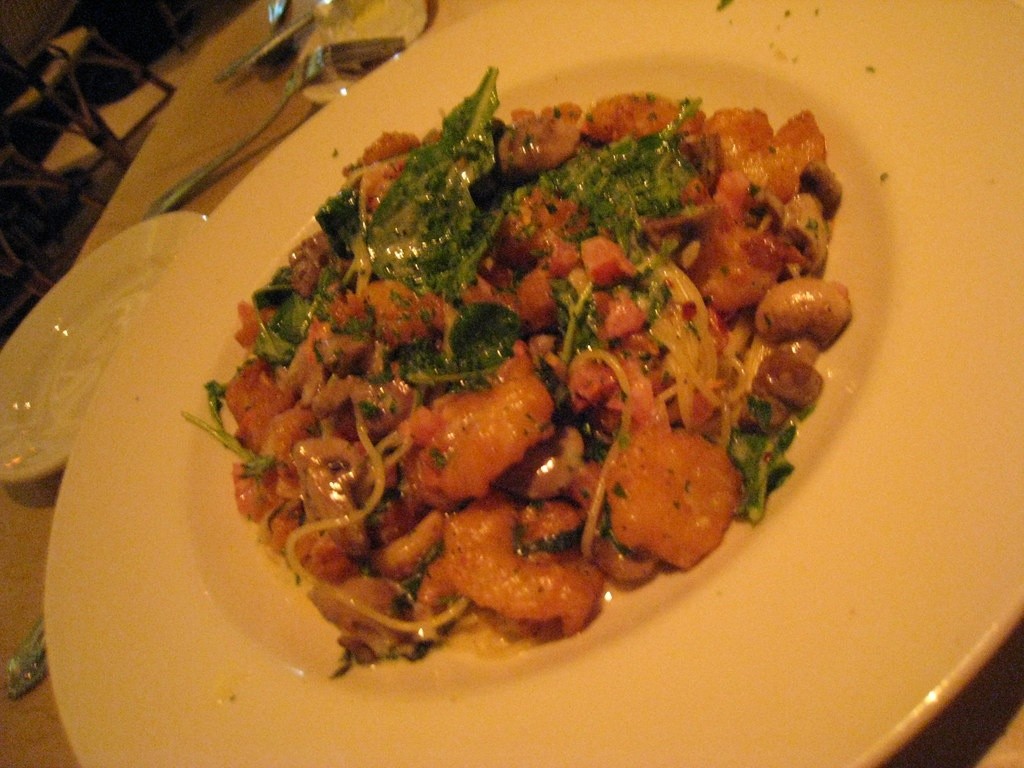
[267,0,287,23]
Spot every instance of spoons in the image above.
[215,0,349,82]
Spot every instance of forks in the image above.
[143,37,406,218]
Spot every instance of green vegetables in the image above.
[180,63,797,555]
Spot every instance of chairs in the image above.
[0,0,176,206]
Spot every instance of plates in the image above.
[298,0,430,105]
[0,209,209,486]
[45,0,1024,768]
